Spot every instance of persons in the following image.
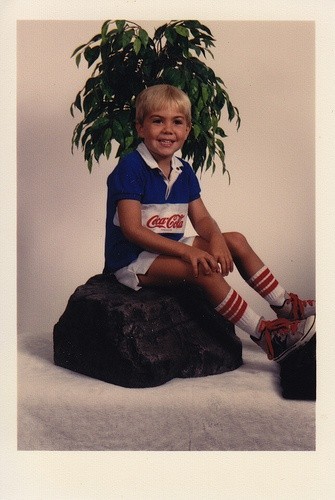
[103,85,316,364]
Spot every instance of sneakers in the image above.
[269,290,316,321]
[250,314,316,362]
